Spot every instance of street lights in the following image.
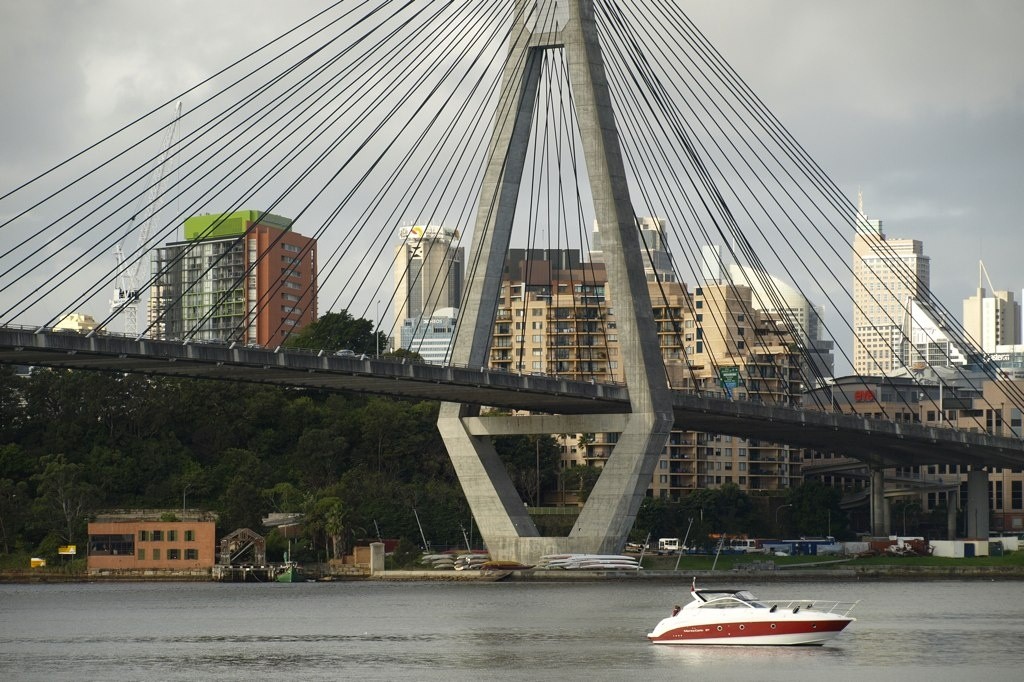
[903,502,921,536]
[376,300,381,359]
[776,503,793,523]
[183,484,192,522]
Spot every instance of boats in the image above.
[646,576,863,647]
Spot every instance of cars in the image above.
[333,349,355,358]
